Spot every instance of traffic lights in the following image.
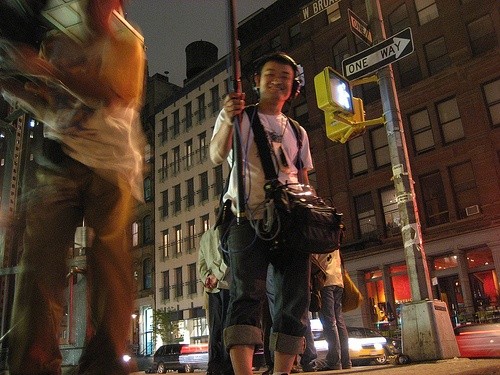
[314,66,355,118]
[325,97,366,143]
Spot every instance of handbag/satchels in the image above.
[213,199,232,230]
[264,180,344,254]
[342,268,363,313]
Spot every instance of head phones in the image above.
[251,52,301,101]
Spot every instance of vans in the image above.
[152,344,209,374]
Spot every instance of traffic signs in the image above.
[342,26,415,82]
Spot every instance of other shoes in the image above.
[262,368,273,375]
[317,365,333,371]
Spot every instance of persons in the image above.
[197,223,229,375]
[312,249,351,372]
[1,0,145,375]
[210,52,313,375]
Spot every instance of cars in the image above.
[310,318,385,367]
